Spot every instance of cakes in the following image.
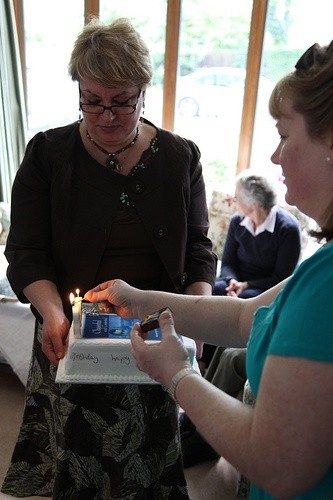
[61,304,197,382]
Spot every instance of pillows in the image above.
[0,202,12,246]
[206,190,310,261]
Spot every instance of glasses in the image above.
[79,80,142,116]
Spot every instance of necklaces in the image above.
[85,124,139,173]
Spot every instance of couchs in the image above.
[0,216,326,389]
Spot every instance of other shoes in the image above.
[179,410,196,432]
[180,431,221,469]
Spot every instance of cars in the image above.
[153,66,276,121]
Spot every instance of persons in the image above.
[0,17,218,500]
[83,39,333,500]
[178,347,248,498]
[213,175,301,299]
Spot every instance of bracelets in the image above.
[167,367,201,406]
